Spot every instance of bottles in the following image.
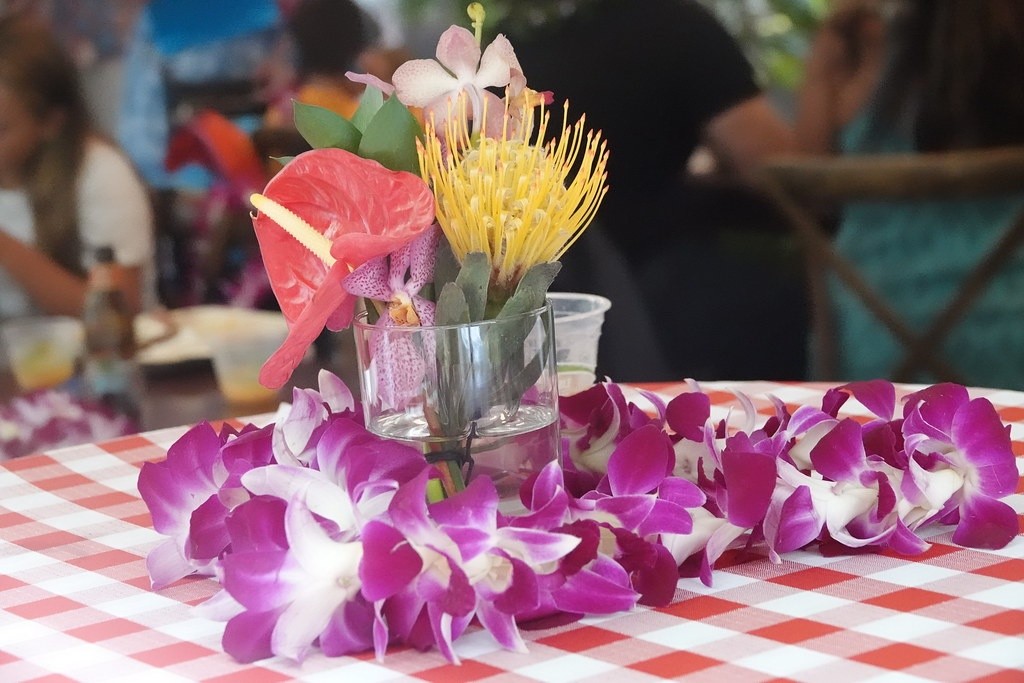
[84,247,145,424]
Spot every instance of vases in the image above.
[349,298,562,517]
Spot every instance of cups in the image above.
[207,325,286,402]
[521,292,615,396]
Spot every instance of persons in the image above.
[790,0,1024,391]
[0,0,815,387]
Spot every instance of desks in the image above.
[1,382,1024,682]
[2,304,315,431]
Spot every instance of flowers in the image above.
[138,368,1020,665]
[248,1,607,390]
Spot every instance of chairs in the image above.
[747,148,1024,390]
